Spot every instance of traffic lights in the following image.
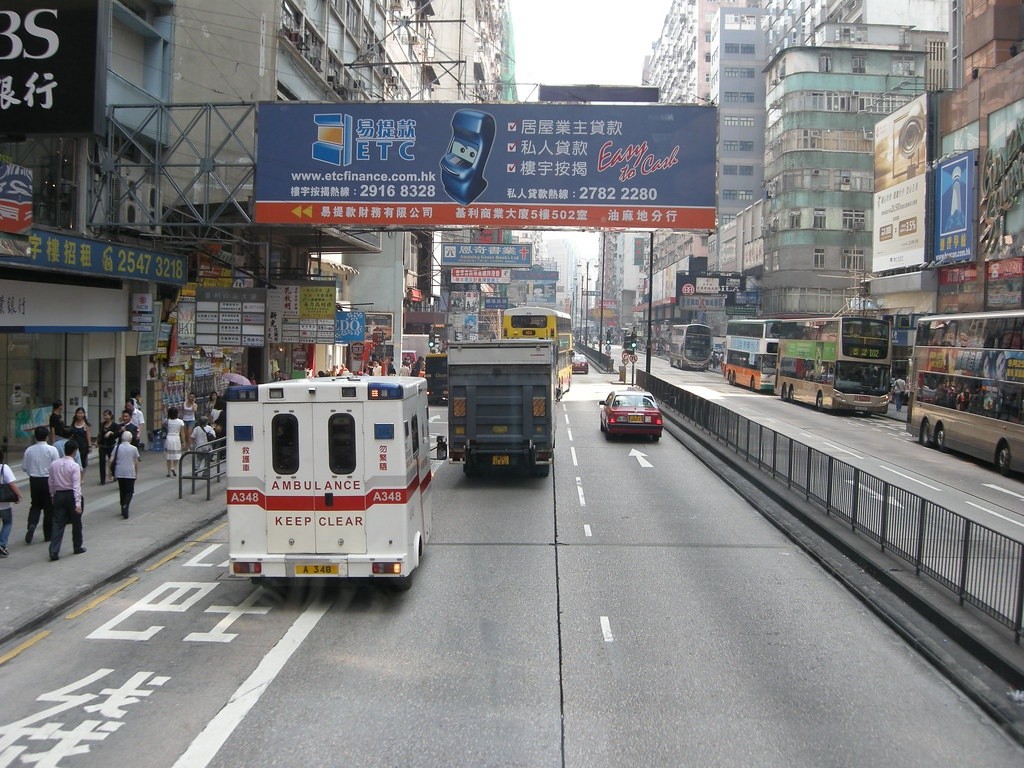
[606,340,611,344]
[632,332,637,348]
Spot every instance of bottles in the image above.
[153,432,164,451]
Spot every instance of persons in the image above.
[49,399,93,489]
[94,388,145,486]
[890,373,905,413]
[226,372,258,397]
[22,425,60,544]
[162,407,186,477]
[180,391,227,476]
[304,353,425,378]
[710,347,724,370]
[270,371,291,383]
[931,386,993,417]
[109,431,140,519]
[0,445,23,558]
[979,350,1018,382]
[49,440,87,561]
[805,365,834,381]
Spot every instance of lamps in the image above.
[927,261,935,269]
[971,66,995,79]
[1009,42,1024,57]
[918,262,927,269]
[864,105,899,109]
[890,87,929,92]
[935,259,945,266]
[882,92,918,98]
[899,81,938,91]
[874,98,910,103]
[857,110,889,116]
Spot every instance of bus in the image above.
[425,353,447,405]
[722,319,783,392]
[905,310,1024,479]
[668,324,713,371]
[500,305,573,402]
[773,316,893,416]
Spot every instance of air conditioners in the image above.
[388,77,399,86]
[110,175,156,234]
[382,67,392,79]
[392,0,403,9]
[352,79,366,92]
[364,43,379,56]
[403,16,411,25]
[844,178,851,183]
[762,73,784,232]
[412,36,419,45]
[812,169,820,176]
[852,90,860,96]
[811,131,819,137]
[854,222,864,229]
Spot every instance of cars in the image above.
[599,391,664,441]
[571,353,589,375]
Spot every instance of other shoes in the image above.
[73,548,86,554]
[166,474,170,477]
[122,505,128,519]
[171,468,177,476]
[0,545,9,554]
[44,537,52,541]
[896,408,901,412]
[50,552,58,560]
[98,482,104,486]
[25,524,35,543]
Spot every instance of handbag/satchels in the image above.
[201,427,215,442]
[900,391,905,396]
[0,463,19,502]
[110,445,119,477]
[160,417,169,439]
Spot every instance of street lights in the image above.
[576,262,599,347]
[569,277,591,345]
[590,230,621,362]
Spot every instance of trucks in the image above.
[225,374,448,587]
[402,350,418,365]
[446,338,557,479]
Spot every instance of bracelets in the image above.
[182,441,185,444]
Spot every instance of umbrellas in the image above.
[223,373,252,386]
